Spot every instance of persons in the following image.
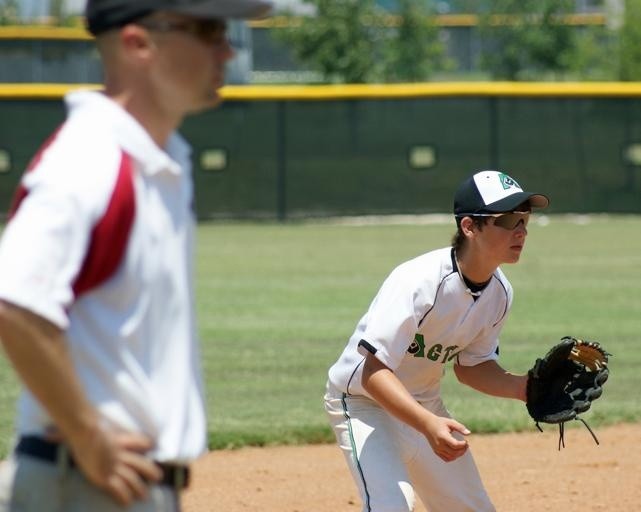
[0,0,276,512]
[323,170,608,512]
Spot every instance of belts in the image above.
[13,433,190,489]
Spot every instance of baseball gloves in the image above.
[526,339,609,423]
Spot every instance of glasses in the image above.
[492,212,531,228]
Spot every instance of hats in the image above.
[454,170,551,215]
[85,0,271,34]
